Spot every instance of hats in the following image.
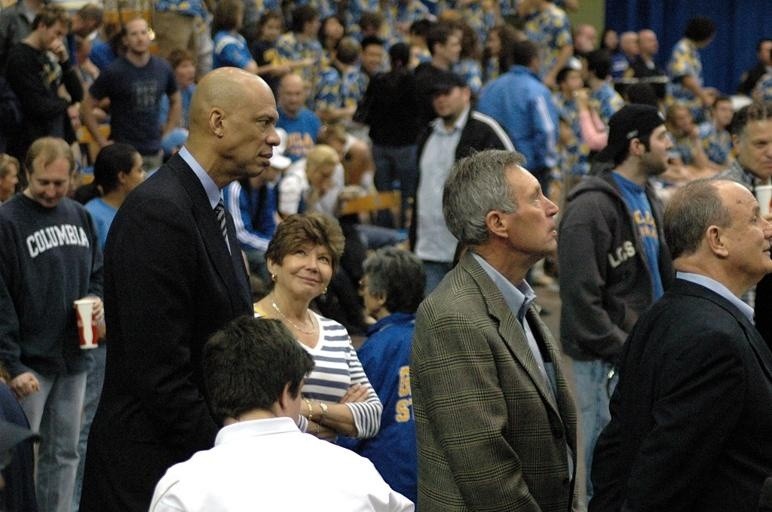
[594,104,666,162]
[422,72,463,94]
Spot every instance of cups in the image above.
[752,184,772,219]
[72,298,102,351]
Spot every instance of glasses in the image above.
[356,280,369,290]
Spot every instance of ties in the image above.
[214,198,232,256]
